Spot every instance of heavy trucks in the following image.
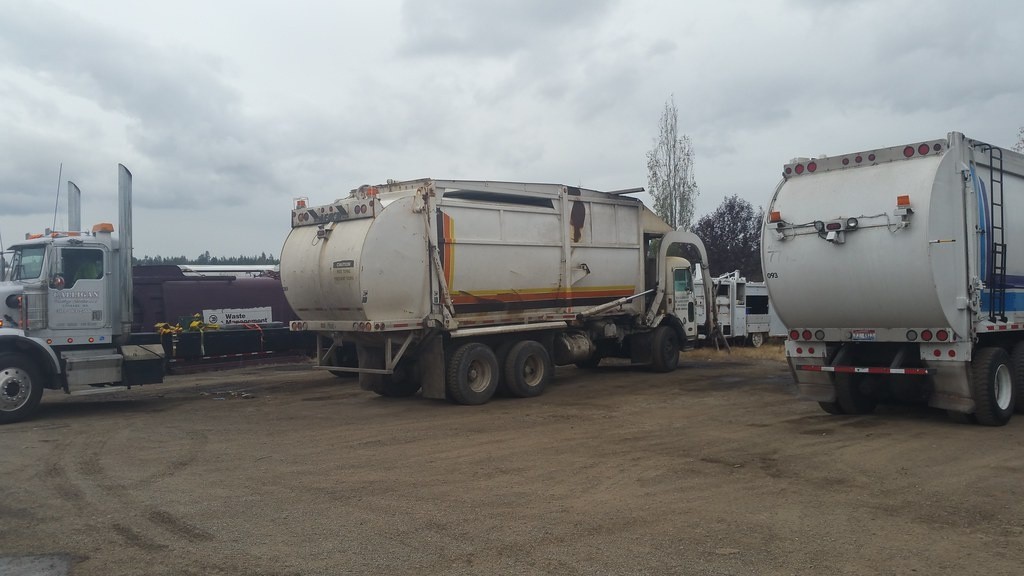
[0,162,344,423]
[277,176,718,405]
[759,132,1023,425]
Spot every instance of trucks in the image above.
[663,249,791,346]
